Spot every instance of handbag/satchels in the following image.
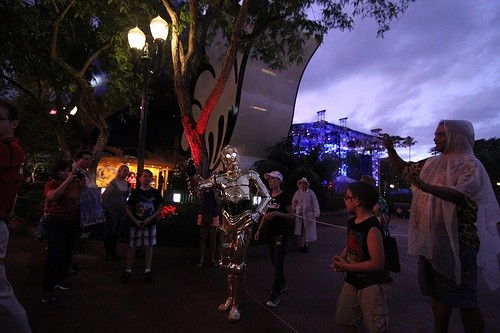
[80,187,106,226]
[384,227,400,272]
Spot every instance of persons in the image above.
[377,120,500,333]
[44,152,91,290]
[329,175,390,333]
[36,161,80,302]
[255,171,294,306]
[184,144,271,321]
[119,169,164,283]
[196,170,219,268]
[291,177,320,254]
[103,165,130,265]
[0,100,32,333]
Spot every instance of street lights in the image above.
[126,14,169,210]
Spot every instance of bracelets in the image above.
[257,228,260,231]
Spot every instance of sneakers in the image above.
[267,298,279,307]
[55,281,70,290]
[271,286,288,294]
[42,292,64,303]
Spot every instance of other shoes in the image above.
[123,270,133,278]
[145,270,152,280]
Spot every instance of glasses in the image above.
[344,194,355,202]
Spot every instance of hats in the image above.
[297,178,309,187]
[264,171,283,182]
